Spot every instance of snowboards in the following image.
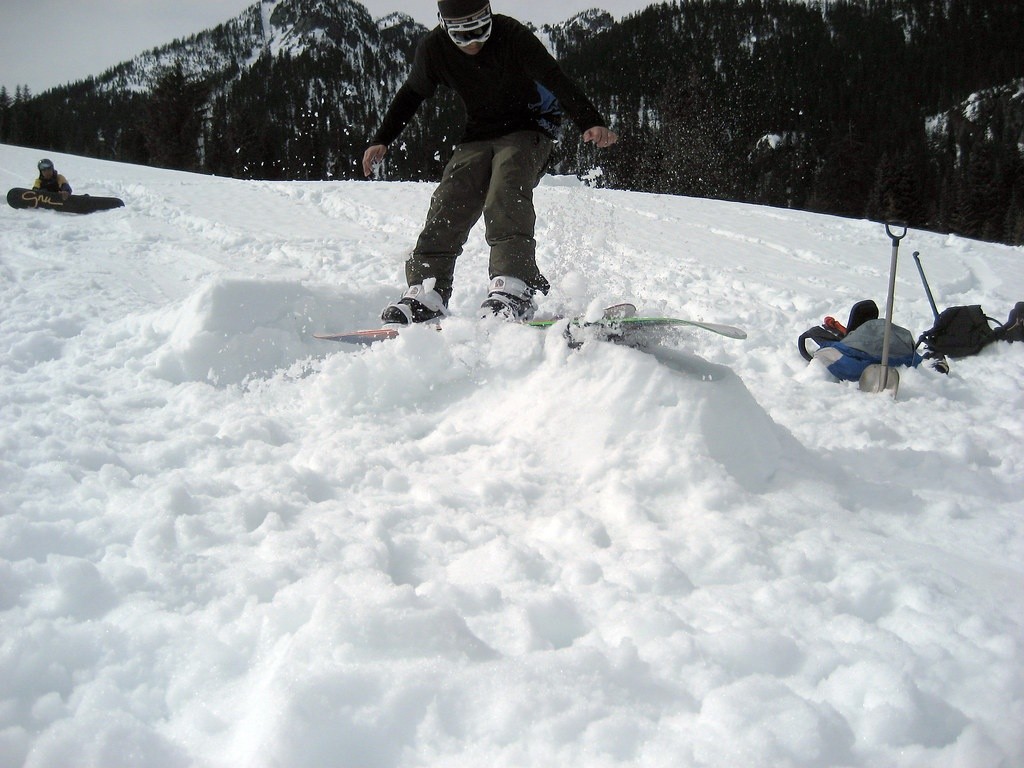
[313,320,749,347]
[7,188,125,215]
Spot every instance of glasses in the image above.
[437,11,493,47]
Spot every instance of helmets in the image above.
[37,158,54,171]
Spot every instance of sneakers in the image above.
[381,286,453,324]
[480,276,538,323]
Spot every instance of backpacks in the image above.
[798,300,1024,382]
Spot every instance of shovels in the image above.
[856,202,925,411]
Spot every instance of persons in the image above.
[32,158,72,201]
[362,0,616,326]
